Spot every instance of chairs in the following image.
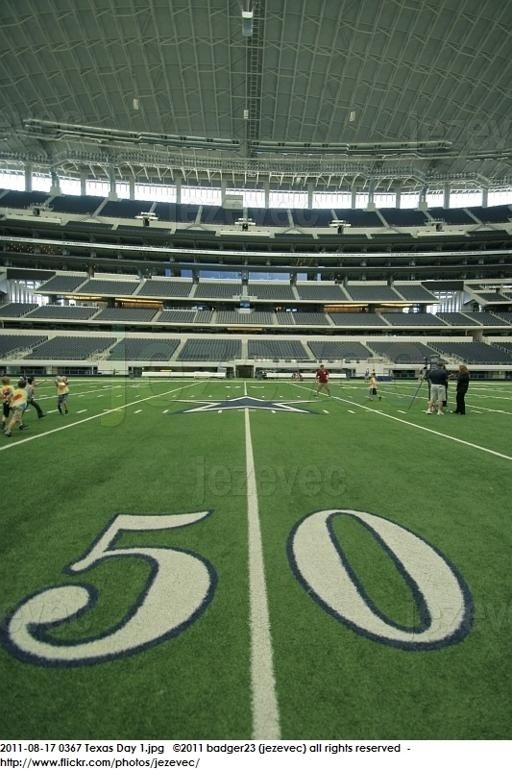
[455,409,464,414]
[19,424,29,429]
[425,408,444,415]
[59,407,68,415]
[37,414,46,418]
[1,421,11,436]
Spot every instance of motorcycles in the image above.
[407,371,431,410]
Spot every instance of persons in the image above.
[425,360,450,416]
[364,368,381,401]
[449,365,470,415]
[0,375,47,437]
[311,364,331,397]
[55,376,70,416]
